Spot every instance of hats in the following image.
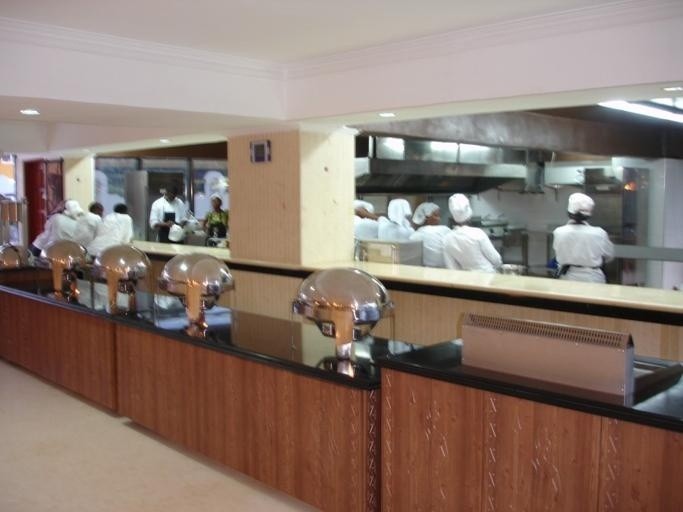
[387,197,412,223]
[209,192,223,205]
[353,199,374,213]
[447,191,472,223]
[63,198,84,216]
[411,201,442,226]
[567,190,595,218]
[167,224,184,242]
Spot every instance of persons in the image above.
[411,202,451,268]
[149,184,189,244]
[442,193,503,273]
[553,193,614,283]
[354,200,378,261]
[202,193,228,246]
[33,200,134,253]
[379,198,415,242]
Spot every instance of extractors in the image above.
[354,134,529,197]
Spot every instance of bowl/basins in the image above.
[500,263,530,276]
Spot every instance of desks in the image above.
[0,277,216,411]
[111,306,427,512]
[0,257,68,281]
[374,337,682,511]
